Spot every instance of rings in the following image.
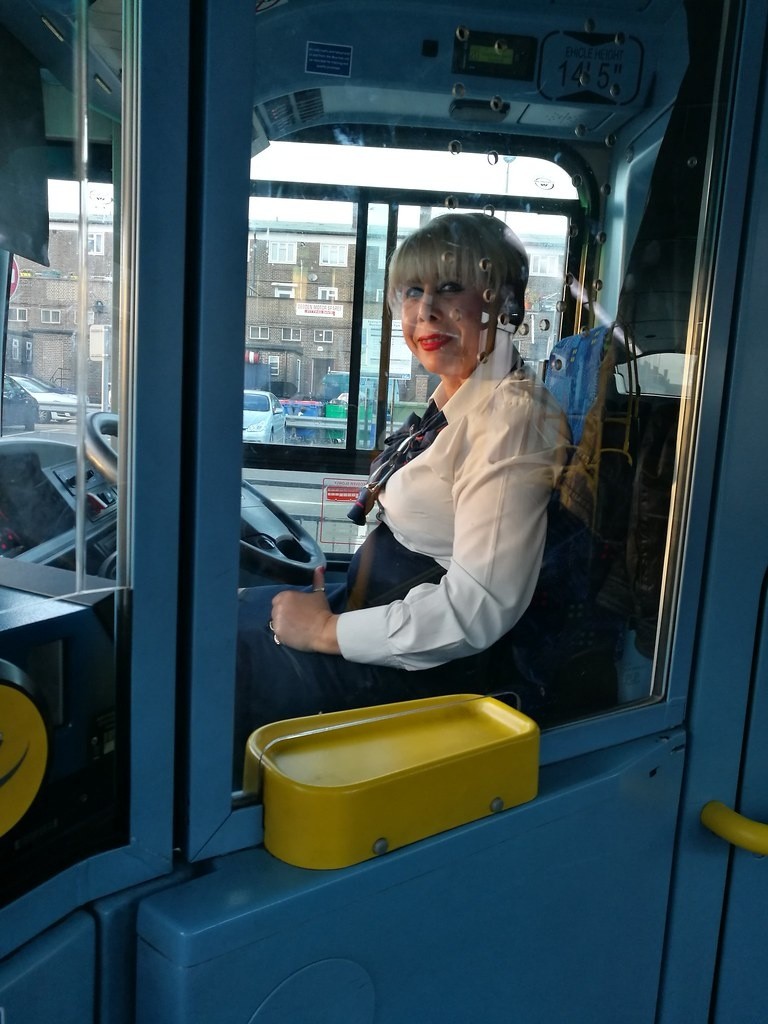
[313,588,325,592]
[272,634,281,644]
[269,621,275,631]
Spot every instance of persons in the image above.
[269,211,577,674]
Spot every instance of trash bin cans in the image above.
[278,400,323,441]
[324,393,372,444]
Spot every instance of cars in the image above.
[5,373,90,424]
[107,382,112,406]
[2,375,38,432]
[242,390,286,461]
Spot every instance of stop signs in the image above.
[7,258,20,299]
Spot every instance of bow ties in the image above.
[346,401,449,526]
